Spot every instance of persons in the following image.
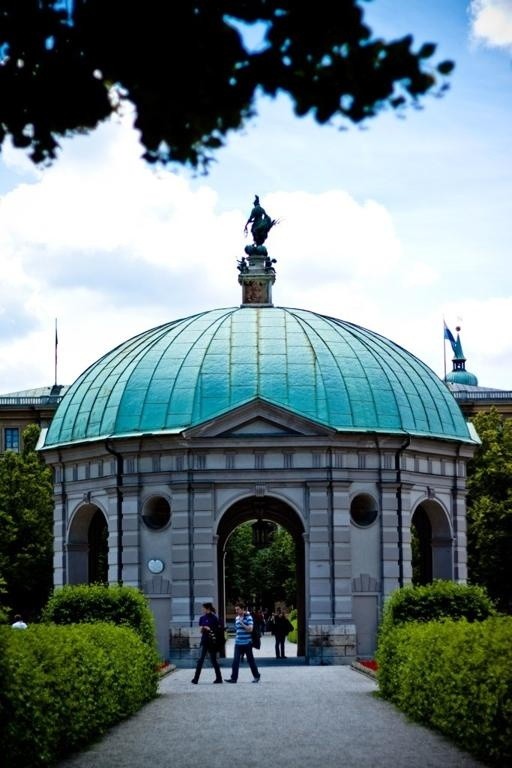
[234,603,276,662]
[237,256,248,274]
[11,613,28,631]
[223,603,261,684]
[265,256,276,274]
[191,603,226,685]
[242,194,269,246]
[273,607,288,660]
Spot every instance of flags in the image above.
[443,319,459,359]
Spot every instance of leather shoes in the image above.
[214,679,222,684]
[224,678,237,683]
[252,677,260,683]
[191,679,198,684]
